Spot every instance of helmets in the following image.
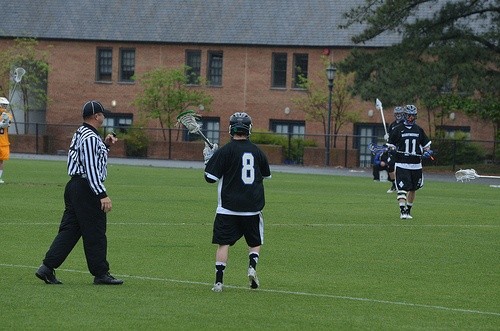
[230,112,251,135]
[393,106,403,120]
[402,105,417,125]
[0,96,9,105]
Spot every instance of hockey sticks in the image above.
[376,98,389,141]
[455,169,500,180]
[6,67,27,113]
[368,143,427,157]
[176,110,214,149]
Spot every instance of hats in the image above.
[83,100,104,118]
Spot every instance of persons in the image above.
[202,112,272,292]
[373,150,391,181]
[35,99,123,284]
[383,105,432,219]
[0,97,11,184]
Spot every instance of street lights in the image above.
[324,64,338,167]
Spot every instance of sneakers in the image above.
[35,265,63,284]
[400,213,412,219]
[94,272,123,284]
[0,179,4,183]
[247,268,259,289]
[387,181,398,193]
[211,283,223,292]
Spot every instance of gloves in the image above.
[0,120,8,129]
[203,139,218,164]
[422,150,433,158]
[2,113,10,124]
